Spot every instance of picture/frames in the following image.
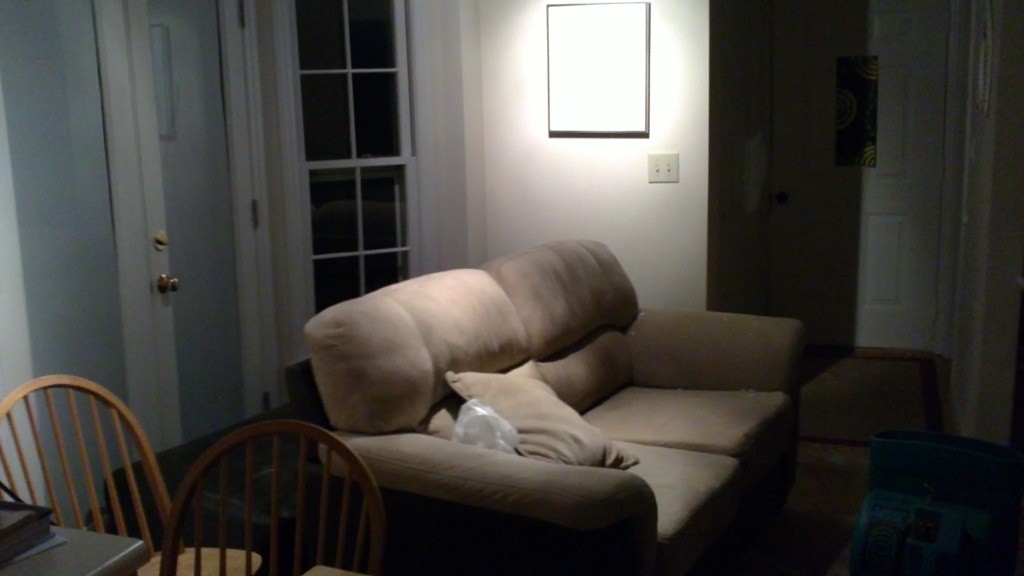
[546,2,650,136]
[148,22,177,140]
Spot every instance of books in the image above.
[0,500,56,564]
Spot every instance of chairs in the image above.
[0,373,262,576]
[158,419,387,576]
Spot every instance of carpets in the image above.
[799,352,945,448]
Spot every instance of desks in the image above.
[0,524,152,576]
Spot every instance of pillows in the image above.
[444,357,639,470]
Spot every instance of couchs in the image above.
[303,238,806,576]
